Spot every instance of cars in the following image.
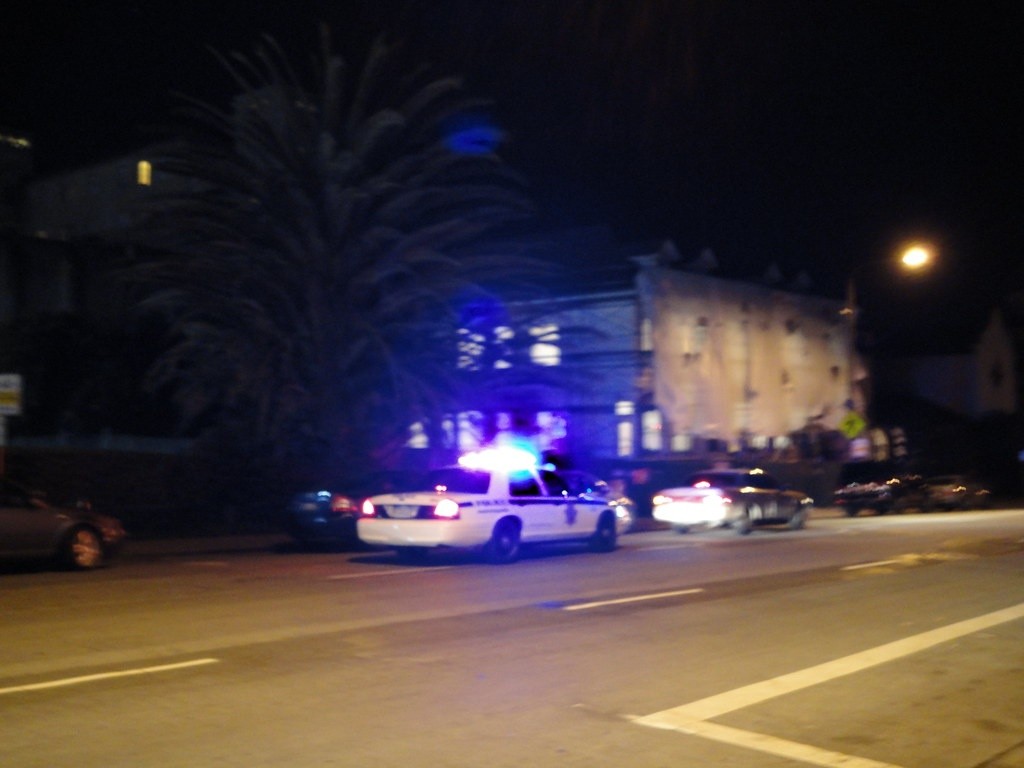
[923,474,990,509]
[357,451,618,564]
[0,479,129,568]
[289,473,424,551]
[555,469,632,535]
[835,472,932,514]
[653,469,813,535]
